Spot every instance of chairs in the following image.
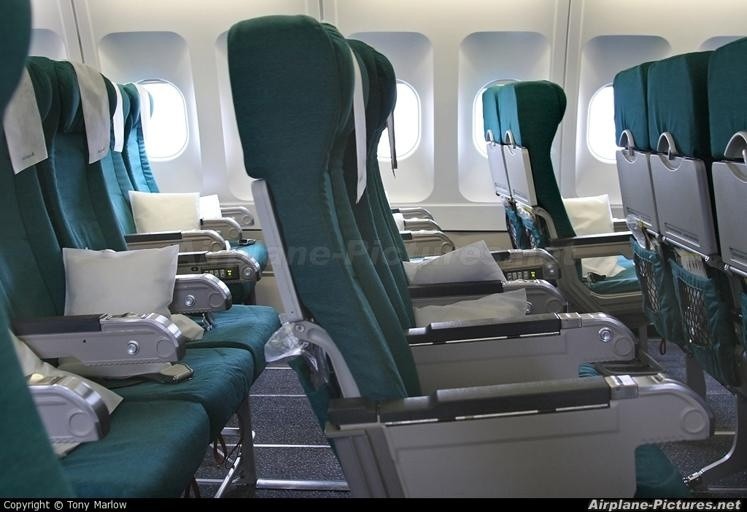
[0,1,745,498]
